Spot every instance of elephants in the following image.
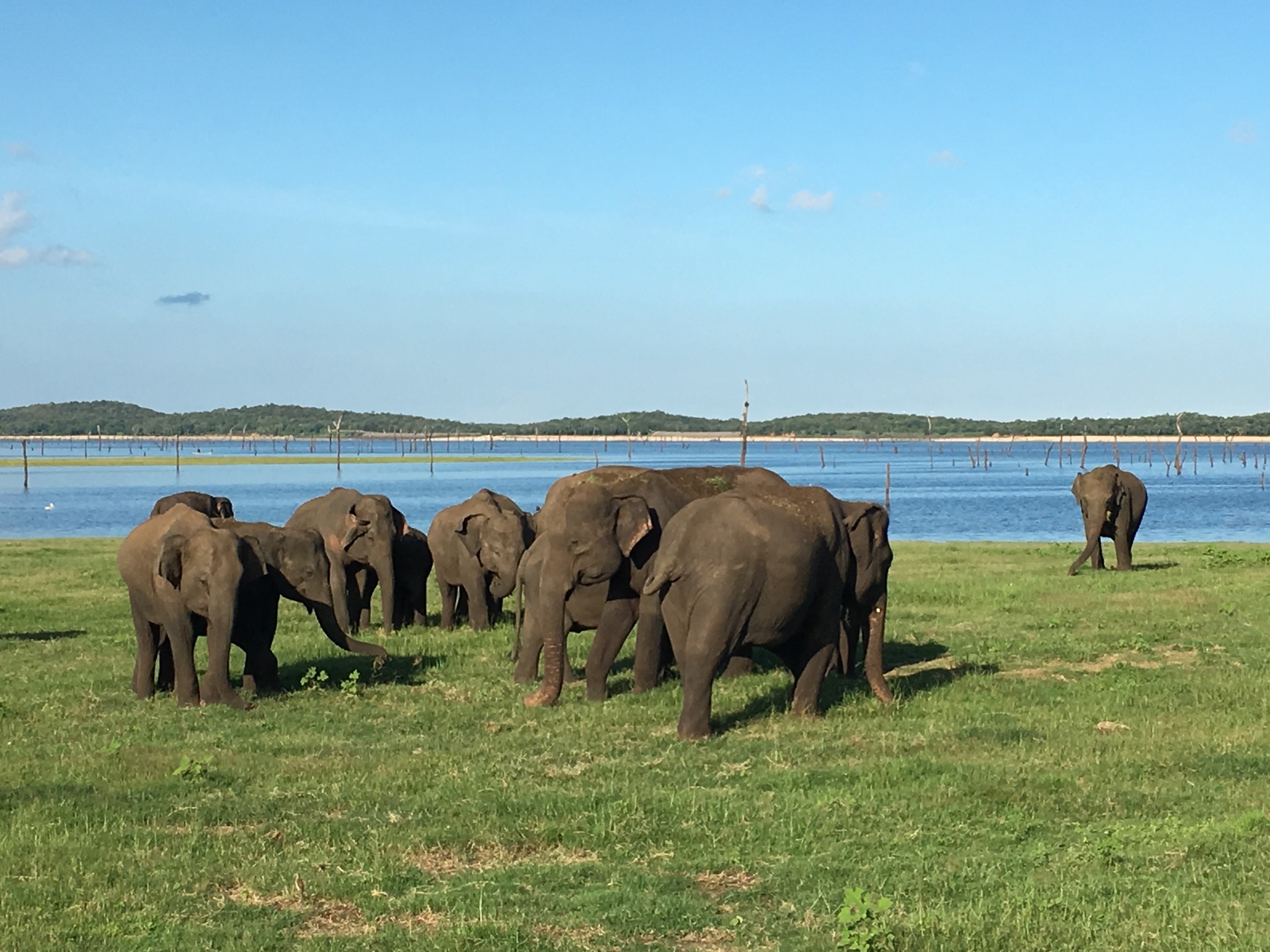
[1066,464,1148,576]
[643,486,894,740]
[115,467,791,711]
